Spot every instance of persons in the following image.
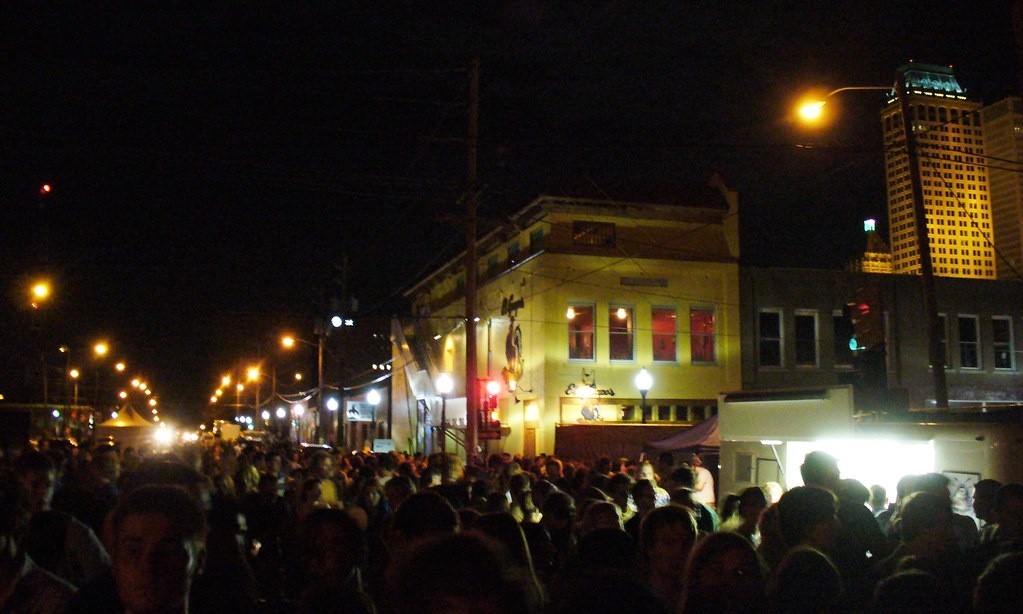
[0,430,1023,614]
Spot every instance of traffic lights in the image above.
[487,382,499,410]
[847,295,874,353]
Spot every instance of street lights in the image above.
[368,388,379,450]
[260,410,269,436]
[249,368,303,434]
[207,375,249,419]
[281,334,325,446]
[293,404,304,446]
[636,368,655,422]
[276,408,286,444]
[32,278,165,428]
[438,372,453,454]
[327,397,336,445]
[795,86,948,408]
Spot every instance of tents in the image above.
[97,401,154,435]
